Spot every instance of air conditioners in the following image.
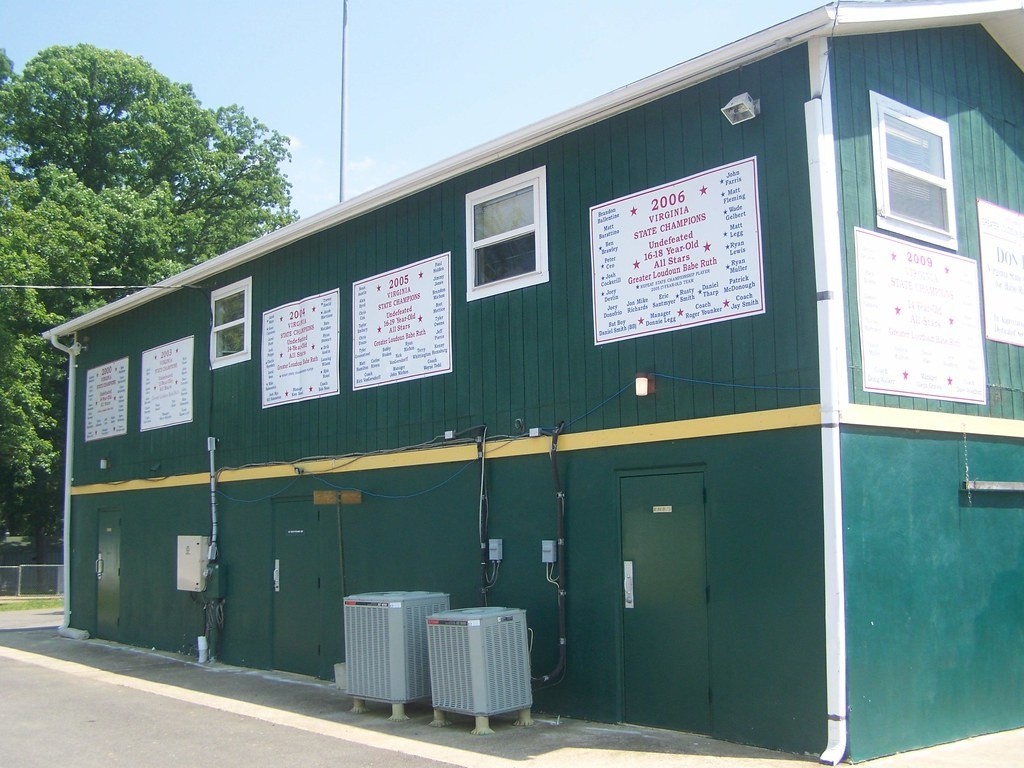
[426,607,533,717]
[343,591,451,704]
